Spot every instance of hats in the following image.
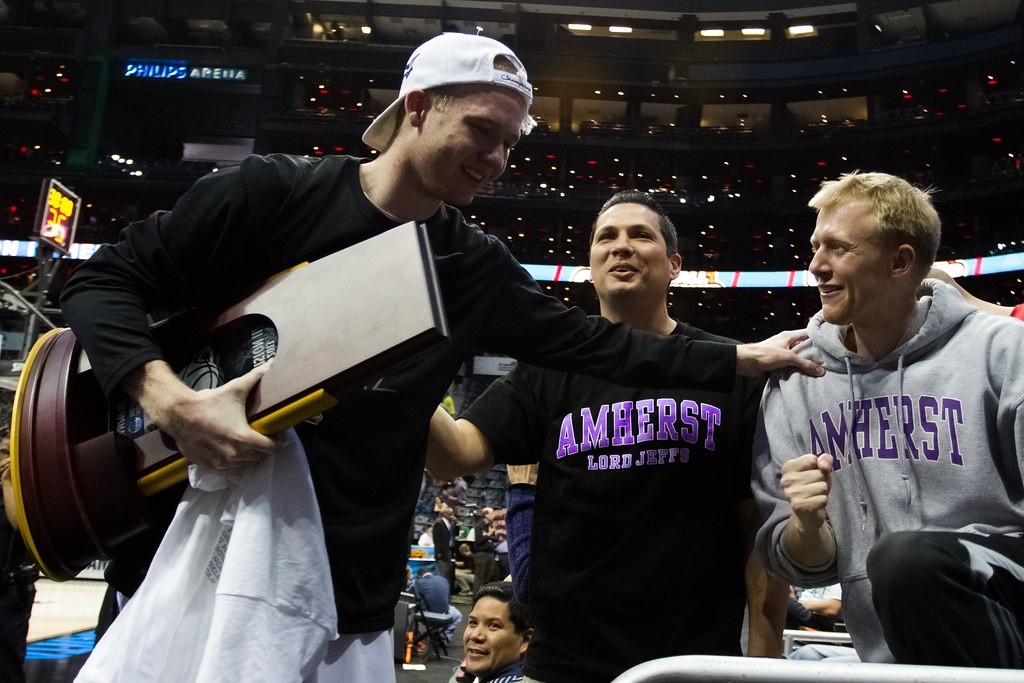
[362,33,533,152]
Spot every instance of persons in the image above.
[0,171,1024,683]
[60,32,827,683]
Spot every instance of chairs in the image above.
[410,580,453,661]
